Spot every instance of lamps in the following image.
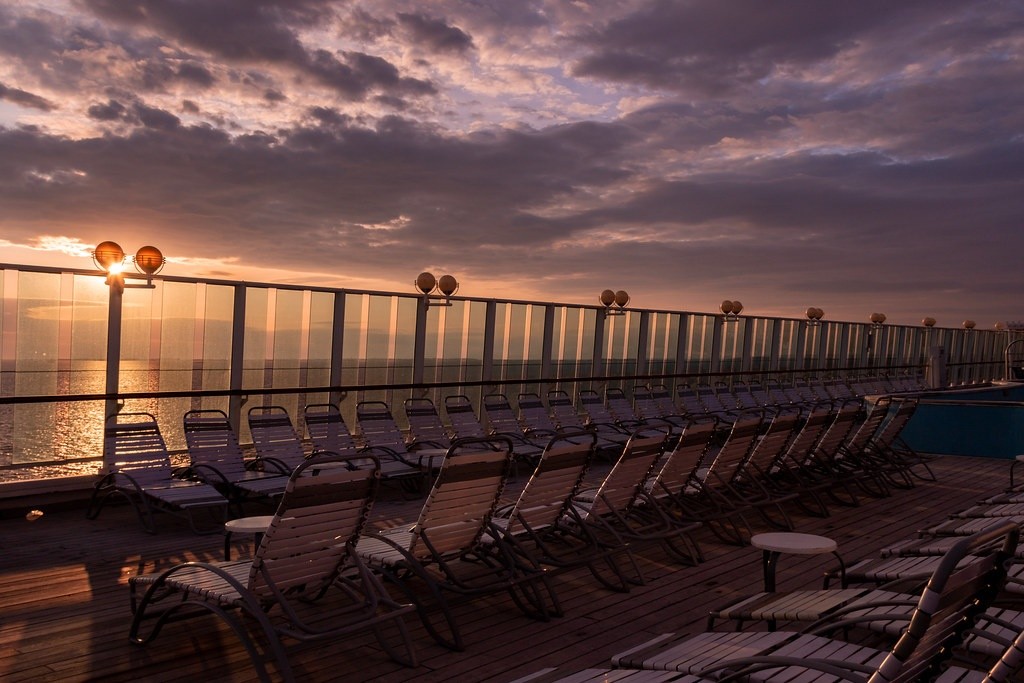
[91,241,165,295]
[415,272,459,311]
[869,312,886,330]
[993,320,1008,335]
[803,307,824,328]
[920,317,936,333]
[598,289,631,319]
[961,319,976,333]
[719,300,743,326]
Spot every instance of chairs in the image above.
[83,367,1024,683]
